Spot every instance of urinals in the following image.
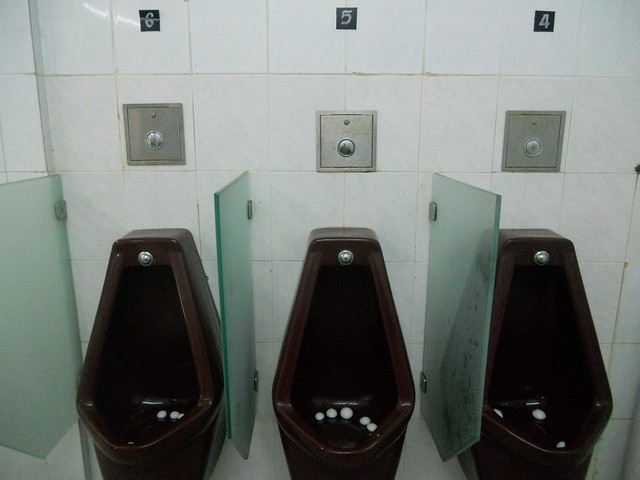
[271,226,416,480]
[76,227,221,479]
[440,229,614,480]
